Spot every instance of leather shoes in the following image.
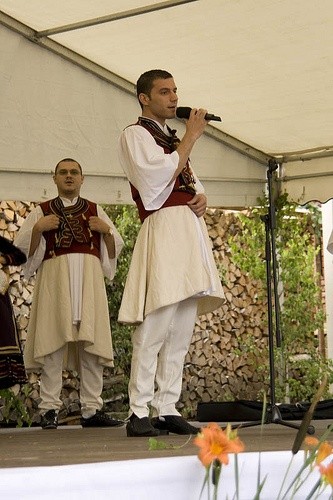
[81,409,124,427]
[40,408,57,429]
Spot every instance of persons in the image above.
[0,236,28,389]
[118,70,225,438]
[12,158,124,429]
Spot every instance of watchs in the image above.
[104,226,113,237]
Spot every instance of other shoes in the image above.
[150,415,198,434]
[126,416,159,437]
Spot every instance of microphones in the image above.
[176,107,222,122]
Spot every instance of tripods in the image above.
[222,157,315,435]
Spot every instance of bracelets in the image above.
[1,254,11,265]
